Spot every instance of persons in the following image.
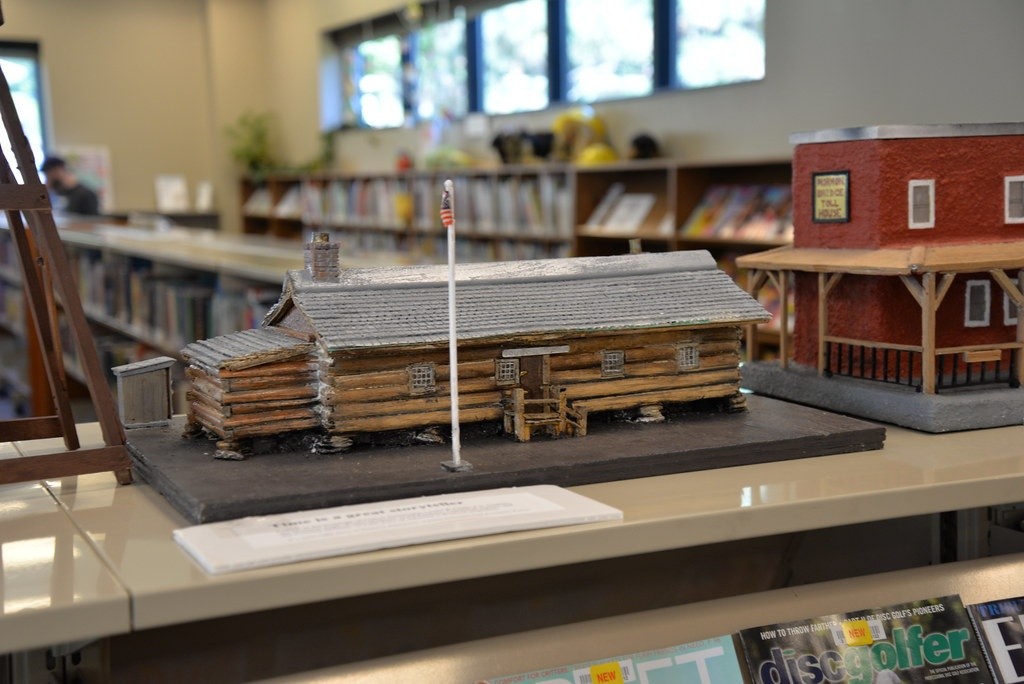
[40,157,98,217]
[630,135,659,159]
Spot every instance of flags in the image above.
[435,189,456,230]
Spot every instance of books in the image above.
[1,234,274,417]
[490,593,1024,684]
[245,178,793,331]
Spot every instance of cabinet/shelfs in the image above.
[0,212,371,423]
[237,156,797,363]
